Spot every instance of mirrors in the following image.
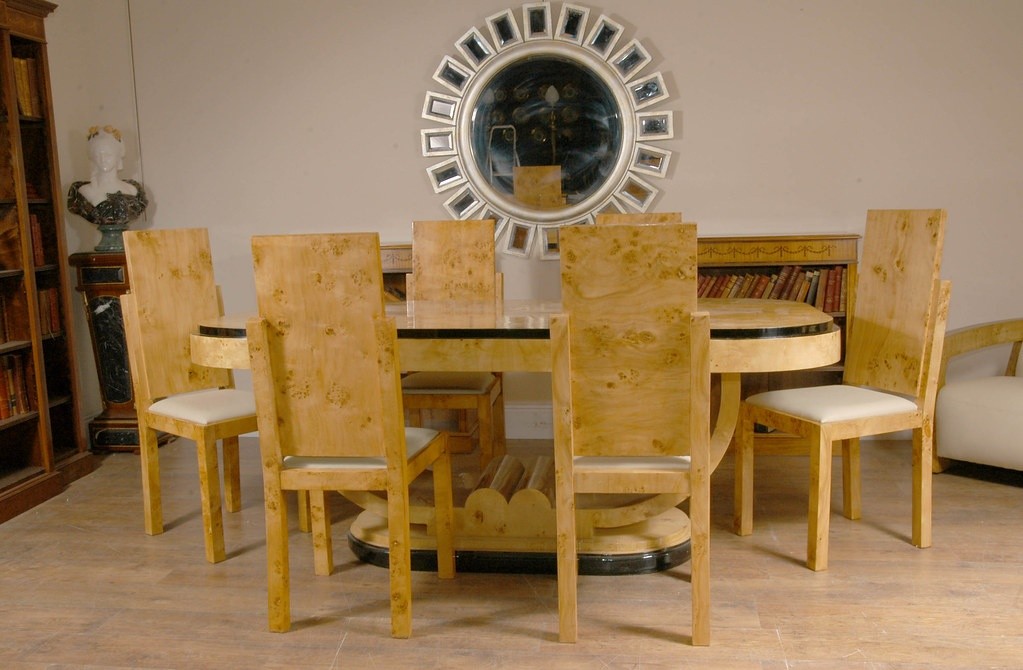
[421,2,674,261]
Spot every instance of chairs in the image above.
[245,232,456,640]
[512,166,562,210]
[400,220,507,471]
[550,210,711,647]
[119,226,258,563]
[731,209,952,573]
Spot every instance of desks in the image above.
[189,299,840,575]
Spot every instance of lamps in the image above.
[545,85,559,165]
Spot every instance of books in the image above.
[29,206,58,267]
[0,350,38,420]
[13,57,44,119]
[38,288,62,335]
[697,265,848,313]
[21,125,52,200]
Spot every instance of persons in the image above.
[67,125,148,226]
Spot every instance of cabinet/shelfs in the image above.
[0,0,95,528]
[696,233,862,456]
